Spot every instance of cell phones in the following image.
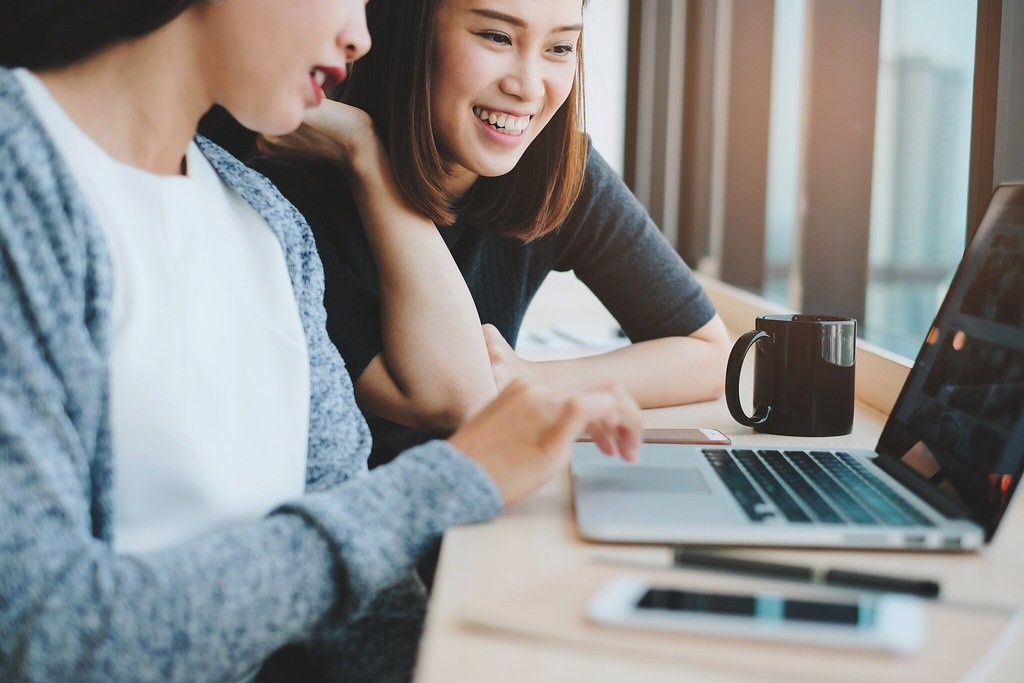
[588,578,923,651]
[575,429,731,441]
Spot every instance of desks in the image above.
[407,333,1024,683]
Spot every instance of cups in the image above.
[725,314,857,437]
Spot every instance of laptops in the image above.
[569,182,1024,551]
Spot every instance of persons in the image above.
[219,0,734,440]
[1,0,644,682]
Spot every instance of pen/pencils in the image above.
[674,546,942,603]
